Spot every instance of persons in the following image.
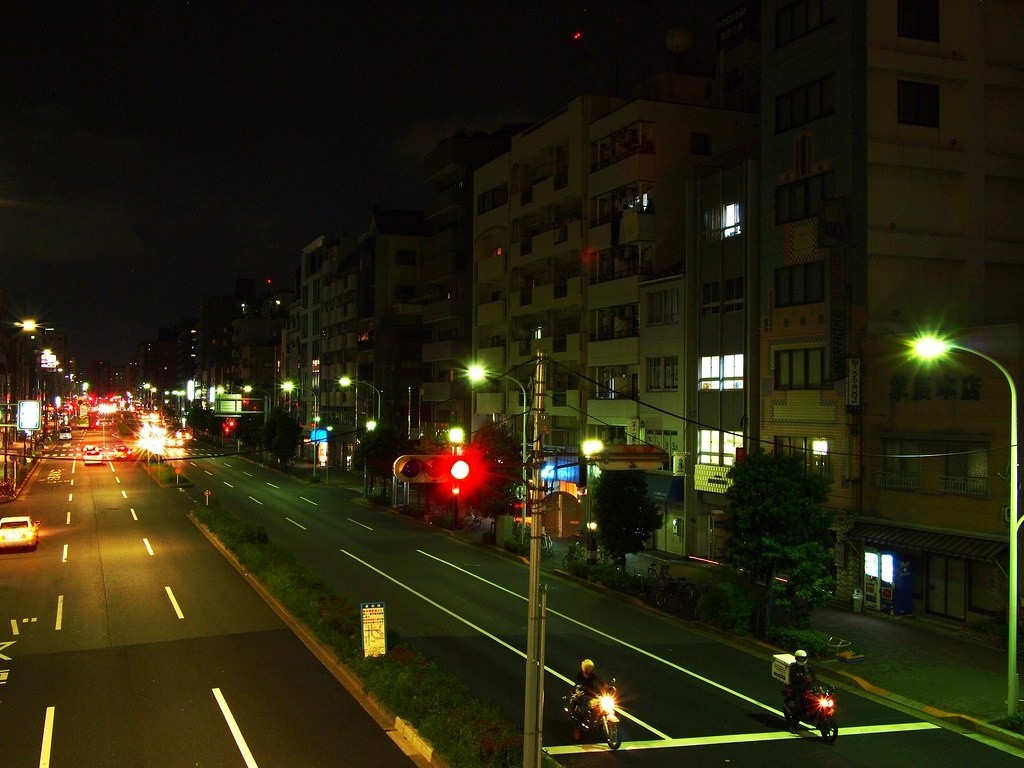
[789,650,822,733]
[571,658,616,740]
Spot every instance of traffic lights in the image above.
[394,455,474,483]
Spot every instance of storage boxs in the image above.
[772,653,797,685]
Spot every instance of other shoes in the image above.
[791,725,796,734]
[574,729,580,740]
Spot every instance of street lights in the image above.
[339,378,381,421]
[449,427,462,530]
[468,366,527,545]
[283,383,316,476]
[912,337,1019,718]
[245,386,271,420]
[583,439,602,565]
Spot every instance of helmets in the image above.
[581,659,594,672]
[794,649,808,666]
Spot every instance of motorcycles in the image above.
[562,677,622,749]
[782,684,838,743]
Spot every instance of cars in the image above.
[81,444,99,455]
[112,445,131,461]
[161,418,191,447]
[0,516,41,551]
[58,411,99,440]
[83,451,103,465]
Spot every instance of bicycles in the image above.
[542,534,580,572]
[464,508,481,527]
[647,560,674,582]
[654,577,695,616]
